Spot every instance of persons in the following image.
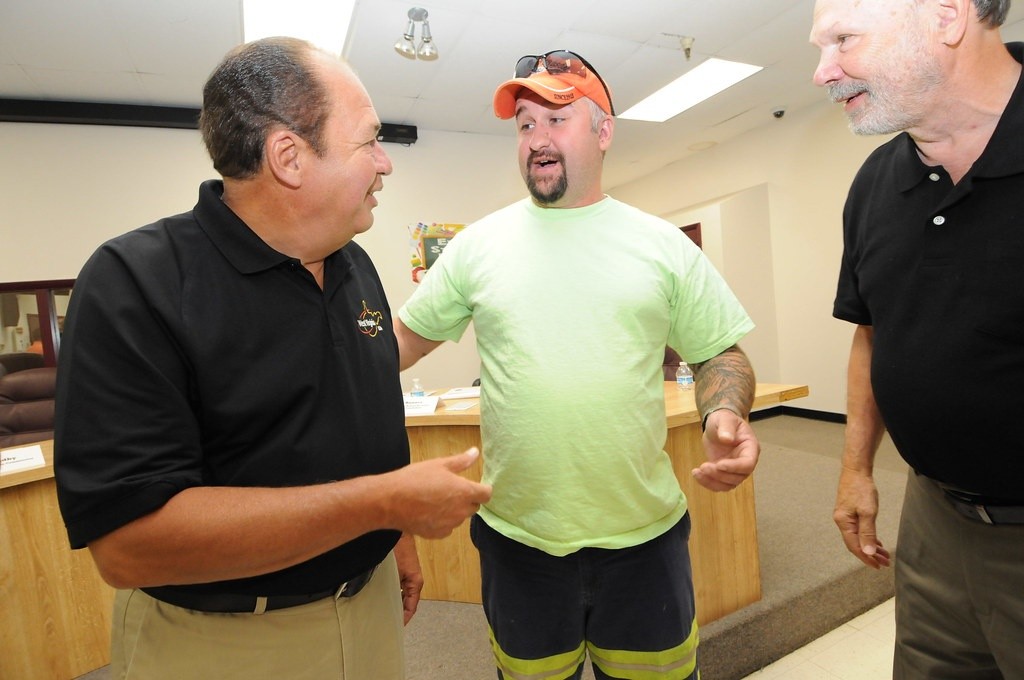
[388,46,761,680]
[807,0,1024,680]
[48,34,496,680]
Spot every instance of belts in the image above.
[942,490,1024,528]
[140,564,379,613]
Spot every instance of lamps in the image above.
[395,7,438,61]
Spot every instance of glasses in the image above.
[514,50,615,116]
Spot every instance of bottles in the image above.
[675,362,694,408]
[409,378,426,400]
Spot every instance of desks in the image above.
[400,381,811,625]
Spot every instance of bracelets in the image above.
[700,403,743,437]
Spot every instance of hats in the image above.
[492,55,612,119]
